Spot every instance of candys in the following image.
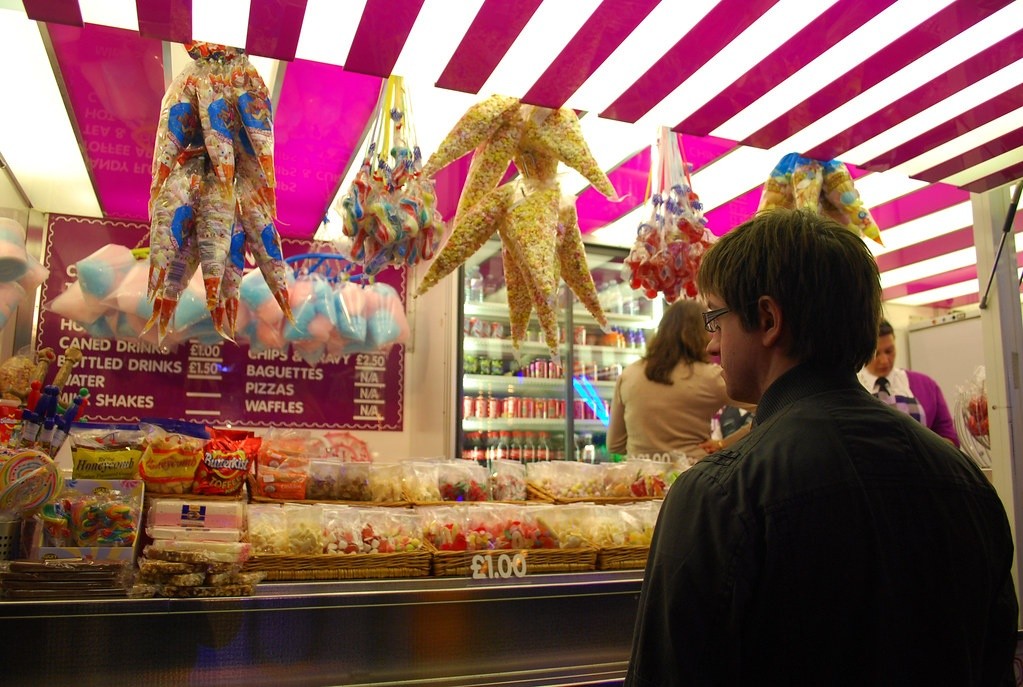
[0,445,138,555]
[302,466,572,555]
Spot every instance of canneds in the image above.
[459,316,646,421]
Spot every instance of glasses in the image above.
[702,298,758,333]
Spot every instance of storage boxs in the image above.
[39,480,144,564]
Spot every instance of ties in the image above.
[875,376,891,401]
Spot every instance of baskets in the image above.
[143,464,665,581]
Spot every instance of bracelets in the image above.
[719,440,723,449]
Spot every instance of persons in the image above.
[605,301,758,467]
[622,207,1023,687]
[855,318,960,448]
